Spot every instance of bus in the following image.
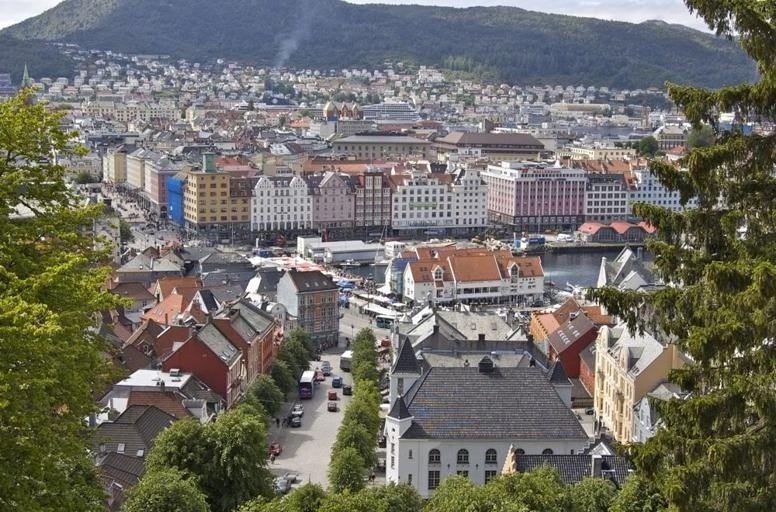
[339,349,353,372]
[375,315,403,329]
[299,370,314,399]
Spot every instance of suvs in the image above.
[291,403,304,417]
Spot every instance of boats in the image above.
[340,259,361,268]
[422,240,456,248]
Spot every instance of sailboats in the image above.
[370,218,390,266]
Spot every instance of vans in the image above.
[333,376,344,387]
[328,391,337,400]
[321,361,331,376]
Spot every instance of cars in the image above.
[507,231,578,248]
[343,385,352,395]
[316,372,324,381]
[292,416,301,427]
[328,403,337,412]
[270,443,283,456]
[273,478,291,497]
